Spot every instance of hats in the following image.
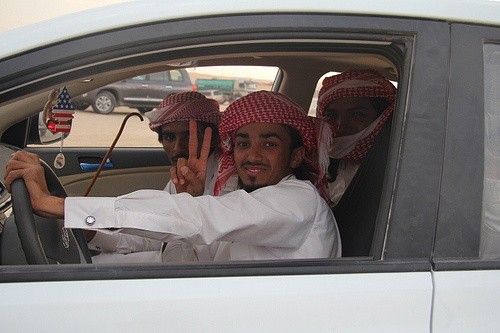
[145,91,218,132]
[214,90,333,204]
[316,68,397,161]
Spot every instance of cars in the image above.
[197,90,224,105]
[68,64,197,113]
[1,0,500,333]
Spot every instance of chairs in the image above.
[330,140,388,257]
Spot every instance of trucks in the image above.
[194,74,259,104]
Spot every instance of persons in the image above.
[307,69,398,258]
[2,89,343,264]
[82,91,239,264]
